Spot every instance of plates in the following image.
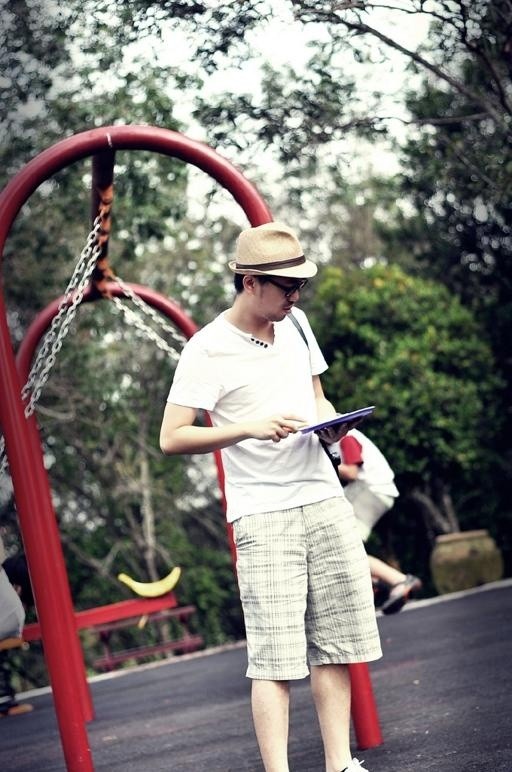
[298,406,376,434]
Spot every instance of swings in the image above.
[3,215,106,652]
[102,269,389,528]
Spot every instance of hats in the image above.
[225,221,319,282]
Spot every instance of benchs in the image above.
[90,604,204,672]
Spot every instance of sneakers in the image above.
[380,573,423,615]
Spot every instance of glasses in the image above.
[261,276,308,298]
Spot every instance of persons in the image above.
[159,222,385,765]
[327,427,423,614]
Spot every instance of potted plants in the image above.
[309,257,507,593]
